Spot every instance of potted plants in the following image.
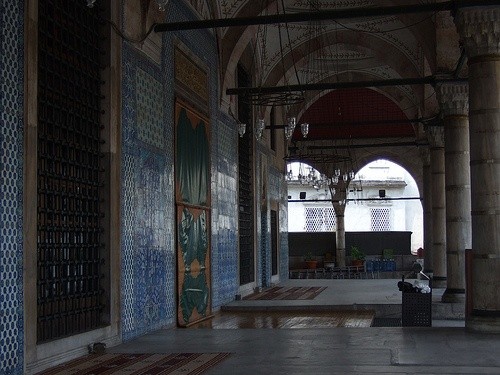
[350,247,365,266]
[305,256,318,269]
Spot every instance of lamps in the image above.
[283,0,363,205]
[237,0,309,140]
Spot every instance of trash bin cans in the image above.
[401,262,432,327]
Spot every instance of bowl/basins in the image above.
[322,261,336,267]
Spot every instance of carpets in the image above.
[243,285,327,300]
[32,352,231,375]
